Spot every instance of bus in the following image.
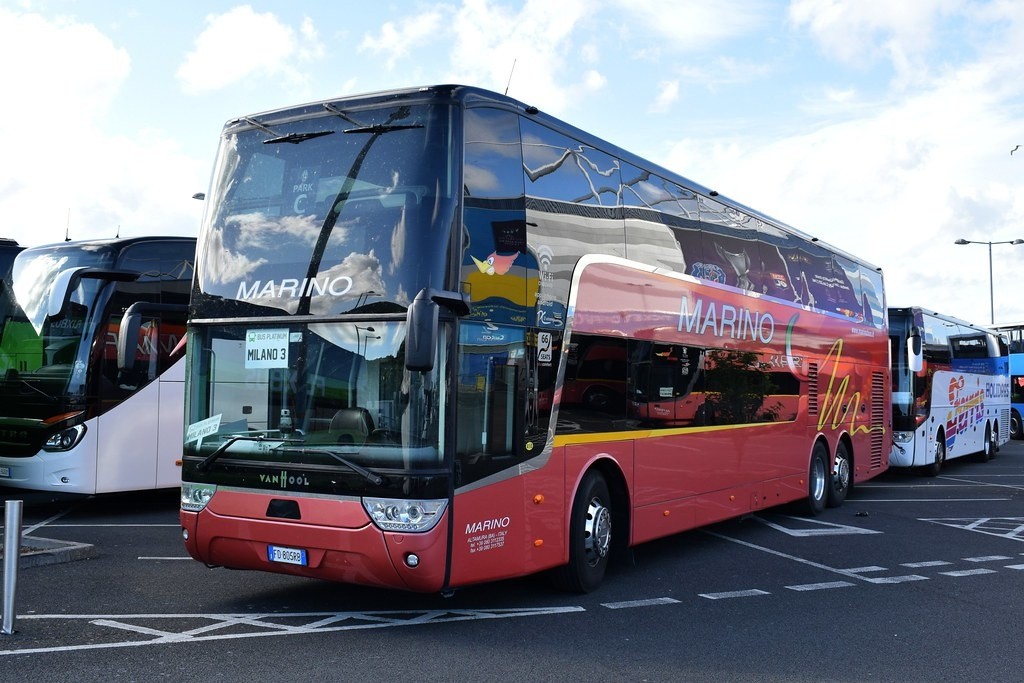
[983,322,1024,441]
[886,304,1011,478]
[116,84,892,596]
[625,339,802,426]
[0,234,272,494]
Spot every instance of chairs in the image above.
[328,407,375,443]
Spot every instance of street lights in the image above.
[954,237,1024,325]
[364,336,381,357]
[354,323,376,355]
[354,290,376,309]
[626,283,653,311]
[363,293,381,307]
